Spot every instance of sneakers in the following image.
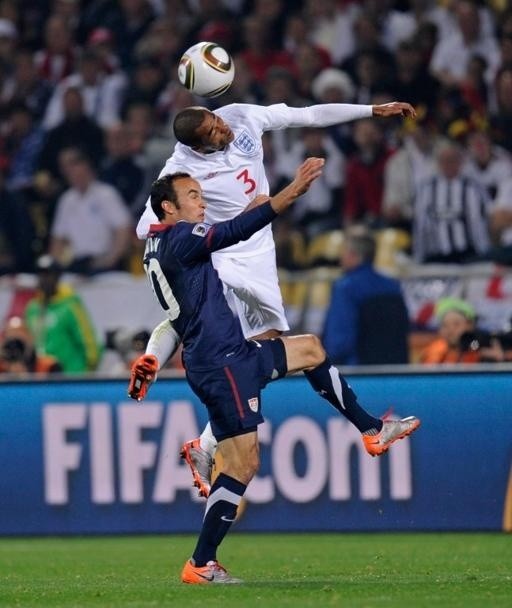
[181,559,243,585]
[127,355,158,403]
[363,416,420,457]
[180,439,211,498]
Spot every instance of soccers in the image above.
[178,41,236,97]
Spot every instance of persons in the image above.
[1,1,511,370]
[143,154,421,584]
[126,99,417,499]
